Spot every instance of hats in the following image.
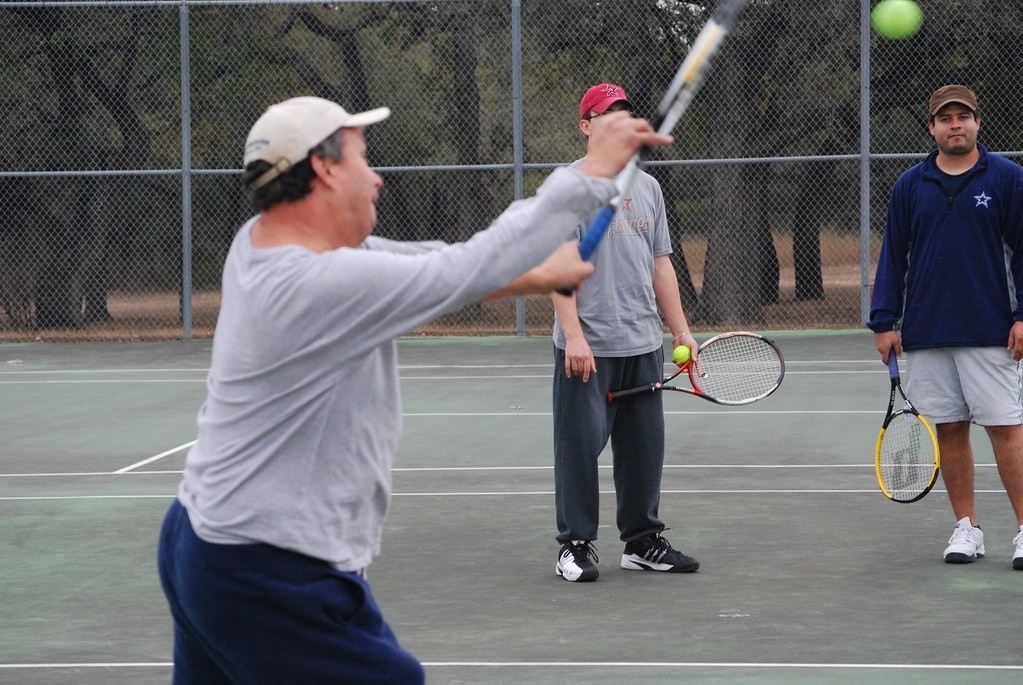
[929,84,977,115]
[581,83,633,120]
[244,96,390,189]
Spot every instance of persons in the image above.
[866,86,1023,570]
[158,95,674,685]
[550,83,701,582]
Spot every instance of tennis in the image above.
[672,344,691,365]
[869,0,925,42]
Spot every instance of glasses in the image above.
[586,108,628,120]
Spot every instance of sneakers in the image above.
[1012,524,1023,569]
[943,517,985,562]
[555,540,599,582]
[621,528,700,572]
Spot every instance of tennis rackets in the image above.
[606,329,787,409]
[573,0,751,261]
[873,346,942,506]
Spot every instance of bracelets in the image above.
[674,331,690,340]
